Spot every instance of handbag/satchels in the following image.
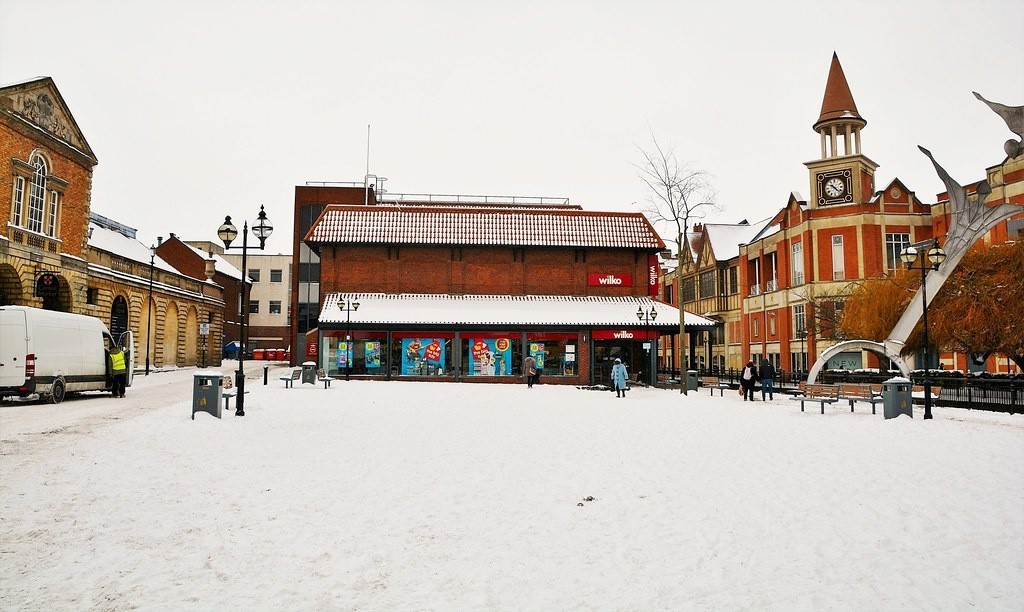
[739,385,744,396]
[529,368,537,375]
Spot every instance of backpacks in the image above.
[743,366,754,380]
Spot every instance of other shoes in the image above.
[763,399,765,401]
[770,397,773,400]
[617,395,620,398]
[750,399,754,401]
[120,394,126,398]
[112,395,120,398]
[528,384,533,387]
[623,393,625,397]
[744,398,747,401]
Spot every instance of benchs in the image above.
[656,374,673,391]
[790,382,884,414]
[911,385,943,408]
[220,375,250,410]
[702,377,728,396]
[316,369,335,388]
[281,369,301,387]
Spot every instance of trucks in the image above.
[0,304,134,403]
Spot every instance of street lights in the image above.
[796,330,808,381]
[900,236,947,421]
[337,300,360,381]
[145,243,158,376]
[637,305,658,389]
[703,337,709,376]
[217,205,274,416]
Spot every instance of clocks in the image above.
[825,178,844,197]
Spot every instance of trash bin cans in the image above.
[276,349,286,361]
[686,372,698,392]
[302,365,317,384]
[286,350,291,361]
[252,349,265,360]
[265,349,276,360]
[882,382,913,419]
[192,375,224,420]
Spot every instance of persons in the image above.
[408,337,422,352]
[610,357,629,398]
[738,360,760,401]
[523,355,537,389]
[759,359,776,402]
[109,346,127,398]
[938,362,946,370]
